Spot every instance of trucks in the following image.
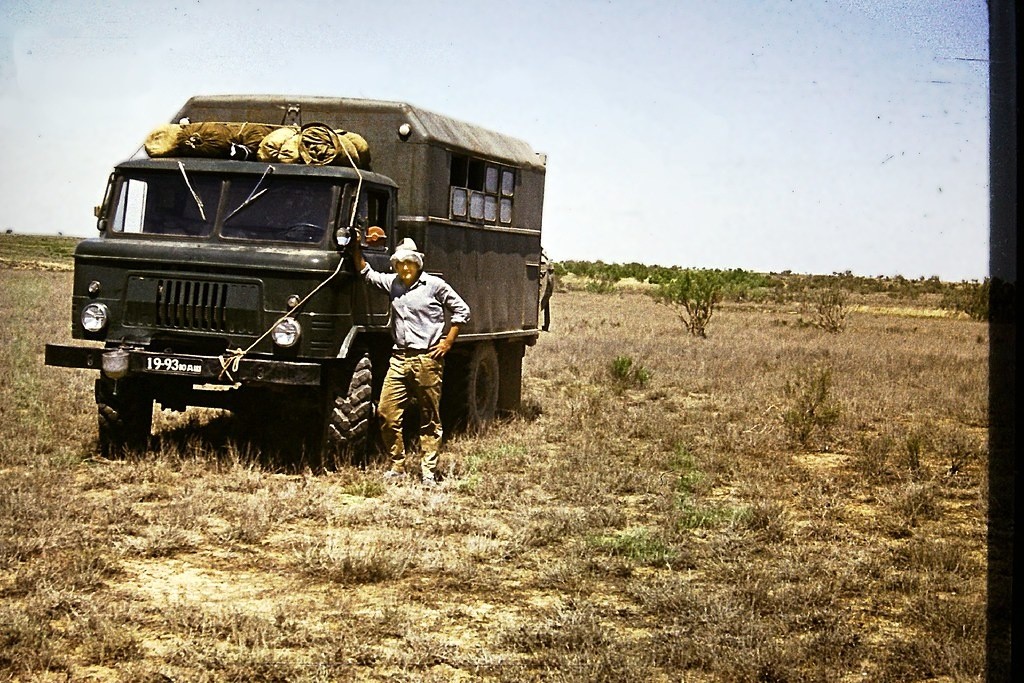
[43,94,549,465]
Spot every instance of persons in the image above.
[352,228,470,483]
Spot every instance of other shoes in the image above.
[421,477,446,489]
[384,471,410,482]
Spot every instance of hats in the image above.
[389,237,424,271]
